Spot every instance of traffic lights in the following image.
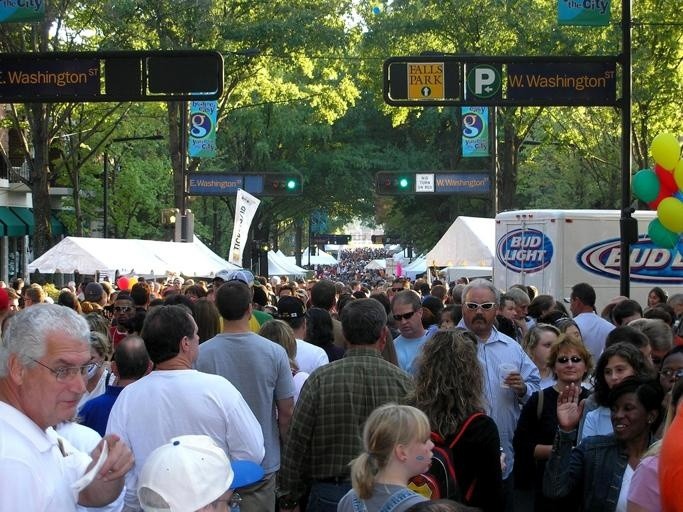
[346,235,351,241]
[374,169,415,196]
[262,172,301,196]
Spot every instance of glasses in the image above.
[16,351,96,383]
[463,301,496,310]
[657,367,683,378]
[391,287,407,292]
[391,311,415,321]
[554,356,582,363]
[210,490,242,510]
[112,305,133,313]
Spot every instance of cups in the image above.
[498,362,519,388]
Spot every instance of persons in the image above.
[0,246,682,511]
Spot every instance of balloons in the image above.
[630,132,683,249]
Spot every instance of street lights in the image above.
[100,133,166,238]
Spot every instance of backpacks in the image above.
[408,410,490,505]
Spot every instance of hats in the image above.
[600,295,630,319]
[276,296,305,318]
[227,268,255,288]
[213,268,232,282]
[133,433,266,511]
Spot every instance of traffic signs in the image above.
[1,54,103,100]
[406,63,446,103]
[336,236,348,245]
[435,172,492,193]
[372,234,403,244]
[186,172,242,196]
[312,235,327,244]
[504,60,615,106]
